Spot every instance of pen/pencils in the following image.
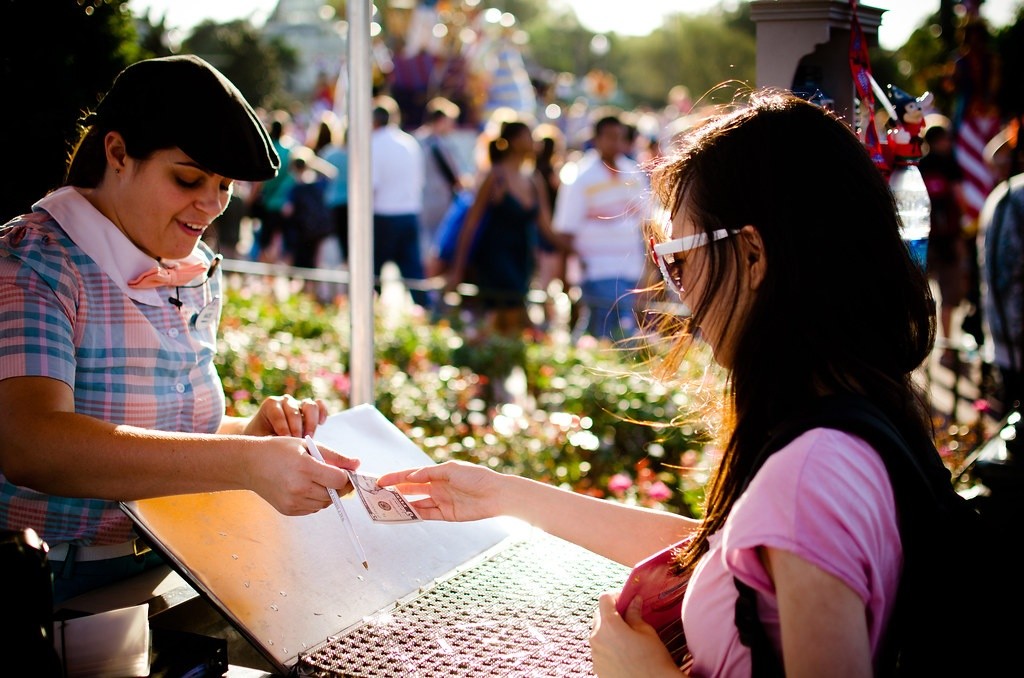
[305,434,369,573]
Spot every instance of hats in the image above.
[114,53,281,181]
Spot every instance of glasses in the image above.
[647,229,741,294]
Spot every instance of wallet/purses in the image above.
[615,533,707,634]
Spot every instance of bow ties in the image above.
[128,261,207,289]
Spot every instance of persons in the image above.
[370,18,1024,678]
[0,55,360,678]
[214,110,349,269]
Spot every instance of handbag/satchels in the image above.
[426,187,488,267]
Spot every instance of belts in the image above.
[45,534,153,562]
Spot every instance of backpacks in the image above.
[731,404,1024,678]
